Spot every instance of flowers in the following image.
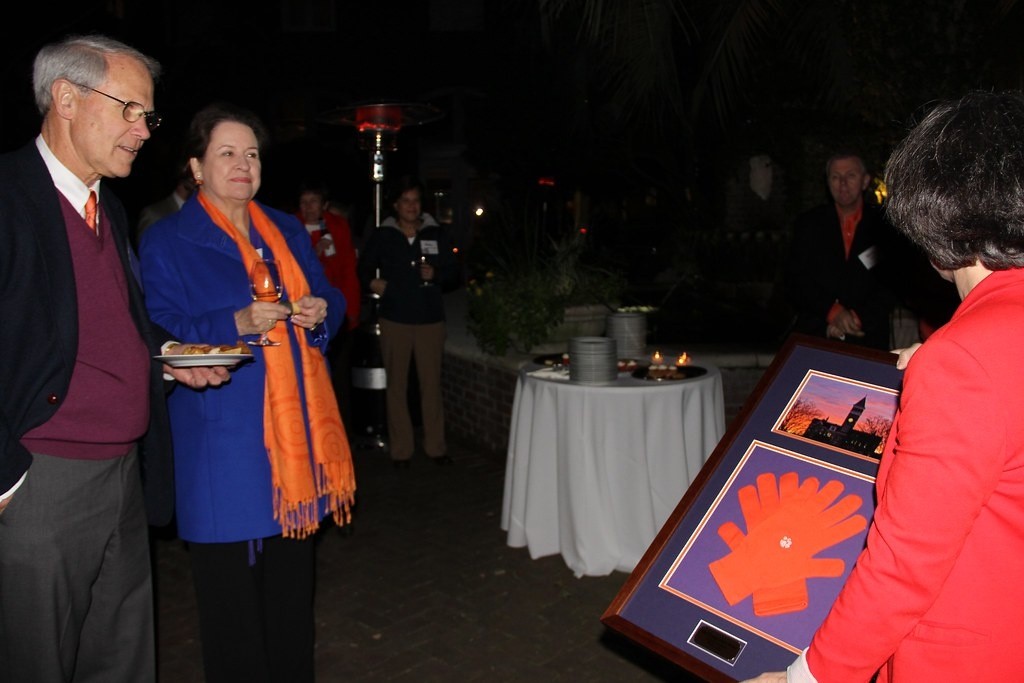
[462,270,565,355]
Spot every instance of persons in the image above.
[361,178,456,471]
[0,33,233,683]
[291,187,361,384]
[136,101,347,683]
[786,151,904,353]
[739,87,1024,683]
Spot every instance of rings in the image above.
[268,320,272,325]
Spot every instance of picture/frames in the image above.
[598,332,906,683]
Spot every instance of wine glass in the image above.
[416,249,434,287]
[247,259,283,345]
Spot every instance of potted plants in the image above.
[513,239,623,353]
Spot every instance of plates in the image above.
[153,354,254,367]
[632,365,707,380]
[569,337,617,382]
[606,313,647,359]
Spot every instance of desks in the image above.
[500,358,726,578]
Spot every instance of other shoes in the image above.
[394,458,409,467]
[426,454,453,465]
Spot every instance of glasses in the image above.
[68,79,160,129]
